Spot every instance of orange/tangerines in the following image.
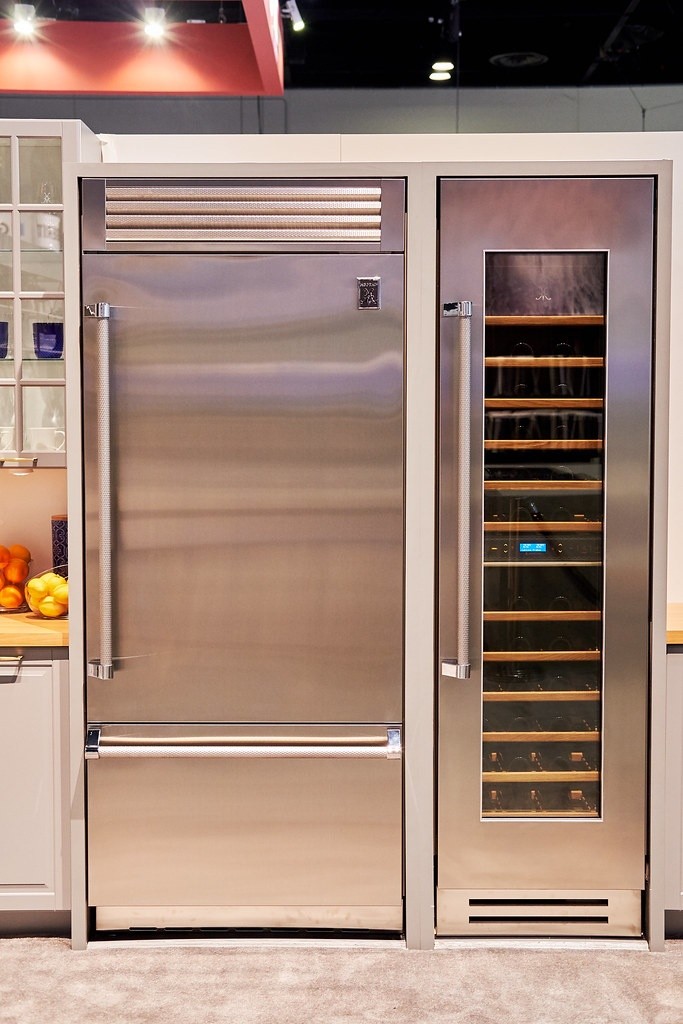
[0,544,31,607]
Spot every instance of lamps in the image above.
[14,3,36,33]
[144,6,167,35]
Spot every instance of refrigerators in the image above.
[79,180,405,936]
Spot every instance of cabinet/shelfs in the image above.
[0,660,71,910]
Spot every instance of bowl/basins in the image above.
[25,587,68,619]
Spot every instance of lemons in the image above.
[28,572,68,617]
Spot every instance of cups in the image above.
[33,322,64,358]
[0,322,9,358]
[0,427,15,450]
[29,428,65,450]
